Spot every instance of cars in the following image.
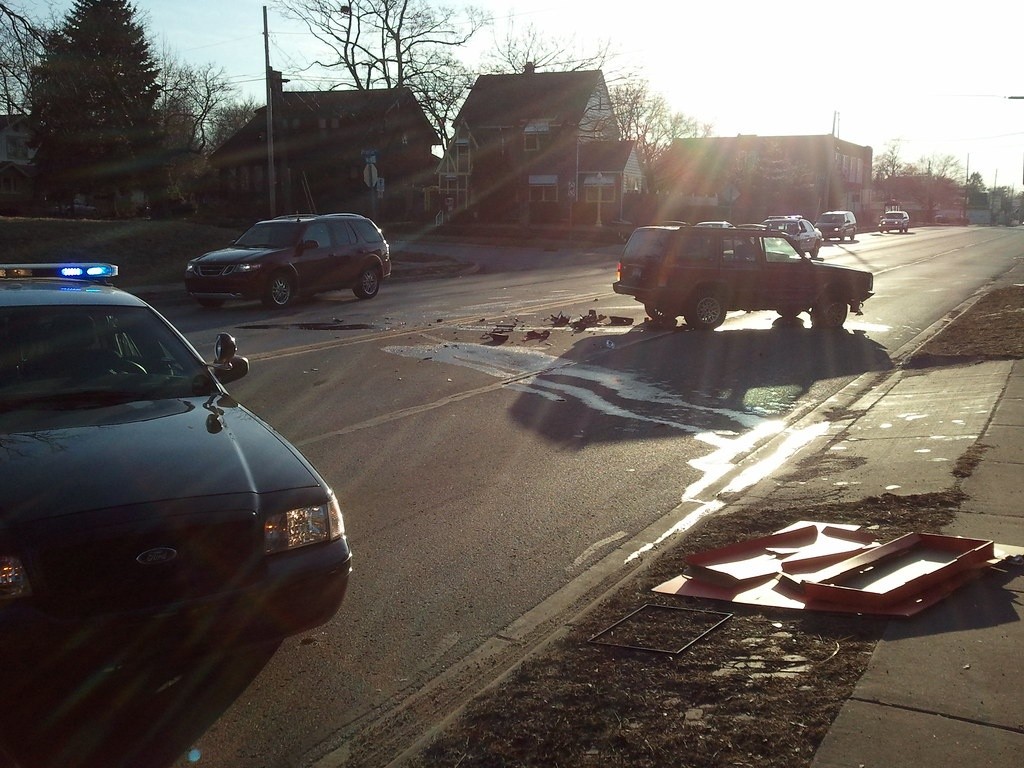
[0,262,352,647]
[760,215,823,259]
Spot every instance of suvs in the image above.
[814,210,858,241]
[613,225,874,331]
[878,211,910,234]
[184,213,391,310]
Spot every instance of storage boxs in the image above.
[684,526,994,607]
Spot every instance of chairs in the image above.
[27,314,124,379]
